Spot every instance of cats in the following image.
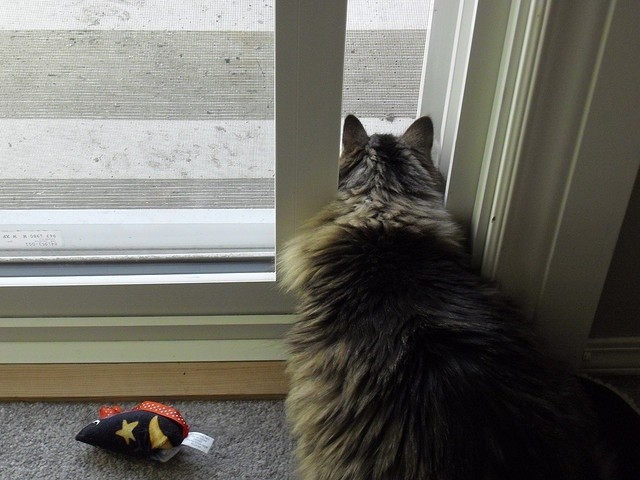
[277,114,640,480]
